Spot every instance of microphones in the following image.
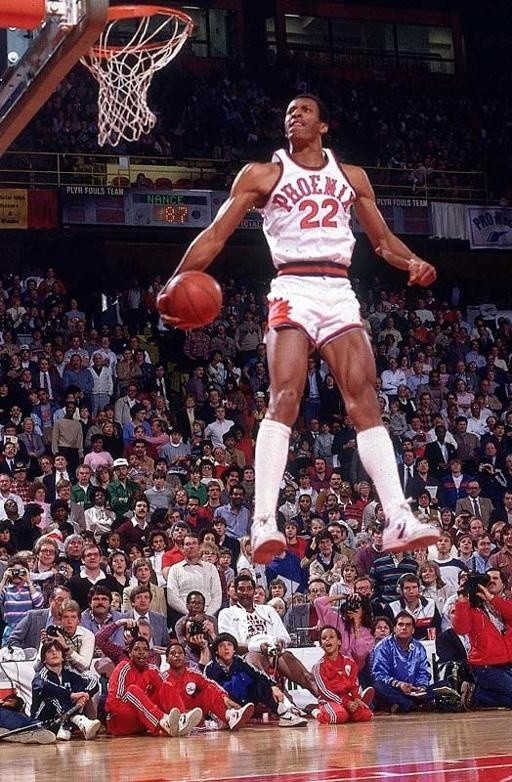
[417,592,423,597]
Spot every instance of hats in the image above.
[113,458,129,468]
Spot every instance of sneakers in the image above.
[460,680,477,713]
[277,711,308,727]
[360,686,375,707]
[381,514,441,554]
[78,719,101,741]
[56,725,73,741]
[163,707,181,737]
[311,708,321,718]
[178,706,203,736]
[227,702,255,732]
[249,514,287,565]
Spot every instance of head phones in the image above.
[395,572,425,593]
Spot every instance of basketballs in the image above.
[165,271,223,328]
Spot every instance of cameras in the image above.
[55,564,67,571]
[12,568,26,576]
[47,625,64,637]
[350,597,361,611]
[130,626,139,637]
[191,623,203,634]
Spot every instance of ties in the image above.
[60,473,64,480]
[10,461,14,476]
